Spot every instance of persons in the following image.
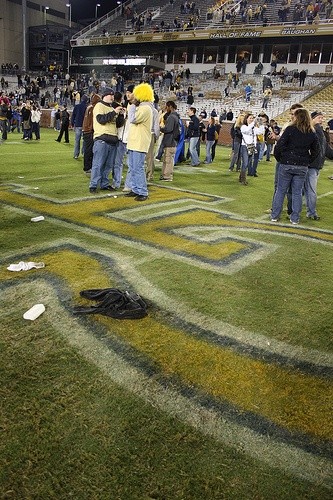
[229,103,333,224]
[223,55,308,107]
[126,0,333,34]
[0,61,234,201]
[104,29,109,38]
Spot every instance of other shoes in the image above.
[124,191,139,197]
[147,182,154,186]
[122,187,132,192]
[135,195,148,201]
[306,213,320,221]
[271,217,280,222]
[100,185,116,191]
[266,160,272,162]
[89,187,97,193]
[263,208,272,214]
[287,214,292,220]
[161,175,173,182]
[289,217,300,224]
[83,169,92,174]
[72,155,78,159]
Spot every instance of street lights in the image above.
[44,6,49,25]
[116,1,122,10]
[66,3,71,27]
[95,4,101,19]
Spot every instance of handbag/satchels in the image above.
[246,142,258,155]
[214,131,218,140]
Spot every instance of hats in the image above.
[91,94,101,105]
[126,85,135,93]
[311,111,322,119]
[102,87,115,99]
[114,91,122,100]
[81,94,88,102]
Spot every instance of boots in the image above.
[239,171,248,186]
